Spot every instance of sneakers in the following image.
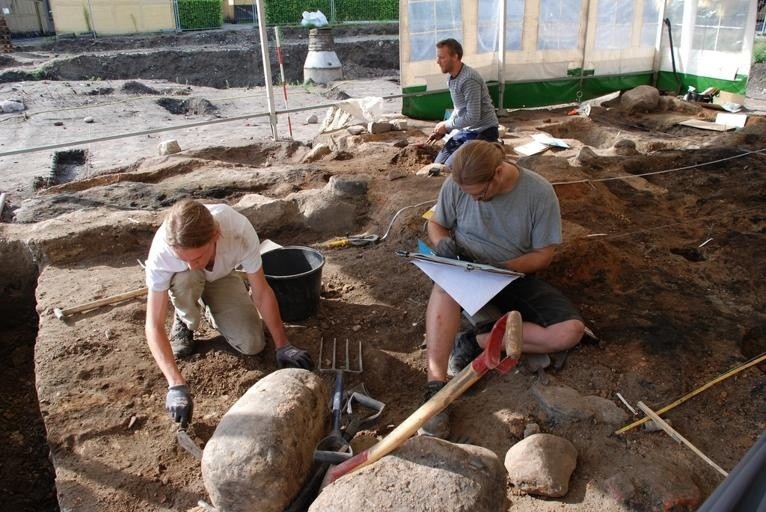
[417,380,451,438]
[447,330,476,379]
[168,311,193,357]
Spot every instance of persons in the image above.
[416,139,585,438]
[145,200,316,428]
[426,38,499,170]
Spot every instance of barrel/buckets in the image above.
[259,246,326,321]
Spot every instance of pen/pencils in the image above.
[452,229,460,261]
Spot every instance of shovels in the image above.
[171,417,203,462]
[666,17,681,94]
[314,310,523,494]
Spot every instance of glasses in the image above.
[472,176,493,199]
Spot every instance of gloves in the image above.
[165,383,193,428]
[274,343,314,372]
[435,238,458,260]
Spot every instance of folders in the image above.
[394,249,525,279]
